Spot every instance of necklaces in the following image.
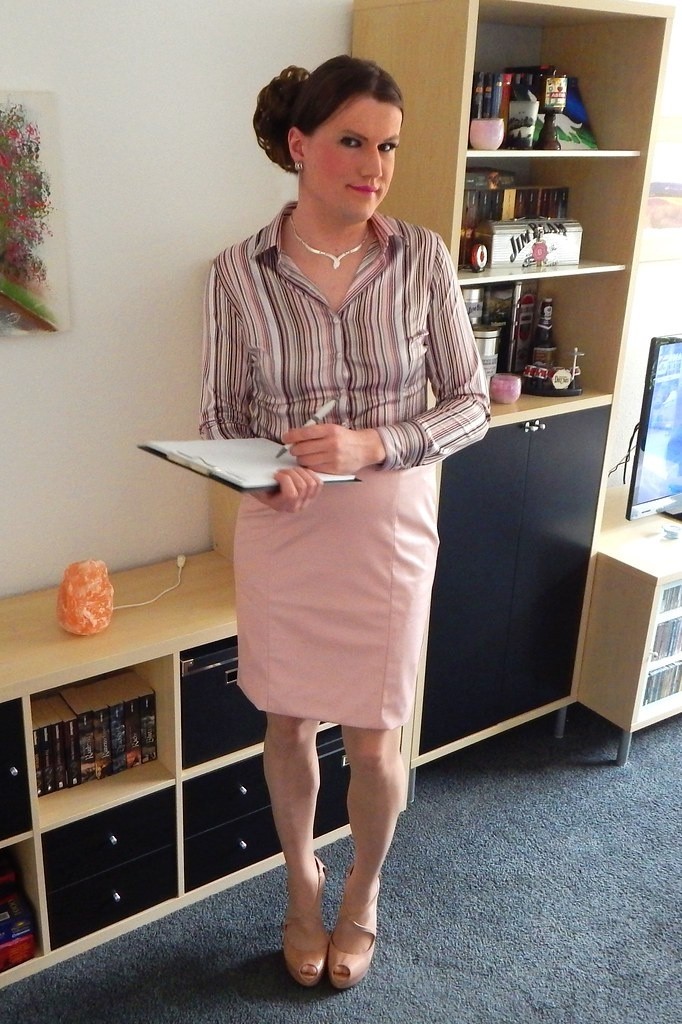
[290,215,370,269]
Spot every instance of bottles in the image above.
[462,285,484,325]
[474,325,499,391]
[530,296,557,365]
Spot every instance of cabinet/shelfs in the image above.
[578,481,682,767]
[0,0,674,990]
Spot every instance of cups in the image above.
[469,117,504,150]
[491,372,521,403]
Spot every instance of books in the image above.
[458,167,569,271]
[31,663,161,798]
[468,65,570,150]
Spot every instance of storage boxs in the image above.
[0,881,35,972]
[312,724,350,839]
[483,279,540,394]
[473,217,584,269]
[180,635,329,771]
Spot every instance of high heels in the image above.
[282,855,327,987]
[328,861,381,989]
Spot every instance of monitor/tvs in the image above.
[625,334,682,523]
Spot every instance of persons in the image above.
[198,54,491,988]
